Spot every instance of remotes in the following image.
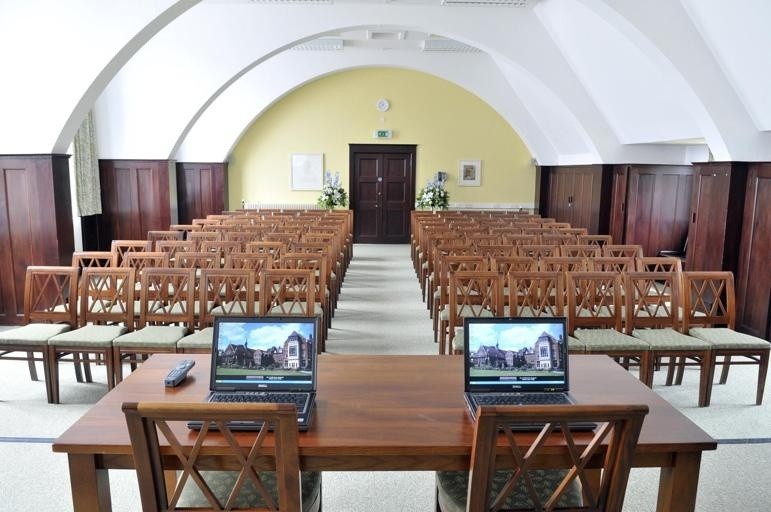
[165,360,195,385]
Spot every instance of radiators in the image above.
[414,202,535,211]
[241,201,320,209]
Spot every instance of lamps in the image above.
[420,40,482,53]
[291,39,344,51]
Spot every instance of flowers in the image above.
[317,170,350,209]
[415,172,450,211]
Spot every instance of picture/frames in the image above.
[289,152,324,191]
[457,159,481,187]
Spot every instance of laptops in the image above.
[463,316,598,431]
[186,316,318,437]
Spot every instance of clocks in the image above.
[377,98,390,113]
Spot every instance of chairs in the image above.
[434,404,649,512]
[121,401,323,512]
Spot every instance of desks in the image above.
[52,353,717,512]
